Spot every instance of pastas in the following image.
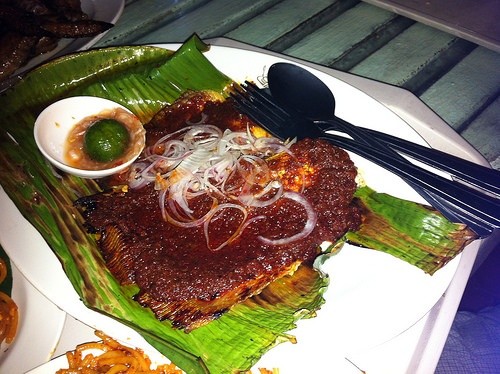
[60,329,182,374]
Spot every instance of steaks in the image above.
[73,91,364,332]
[0,0,114,81]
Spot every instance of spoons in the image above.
[266,61,493,239]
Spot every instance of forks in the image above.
[227,80,500,229]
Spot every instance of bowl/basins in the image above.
[33,96,146,177]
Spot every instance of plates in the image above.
[1,248,68,374]
[22,346,357,374]
[0,0,126,77]
[0,44,461,345]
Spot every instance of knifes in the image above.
[249,87,500,199]
